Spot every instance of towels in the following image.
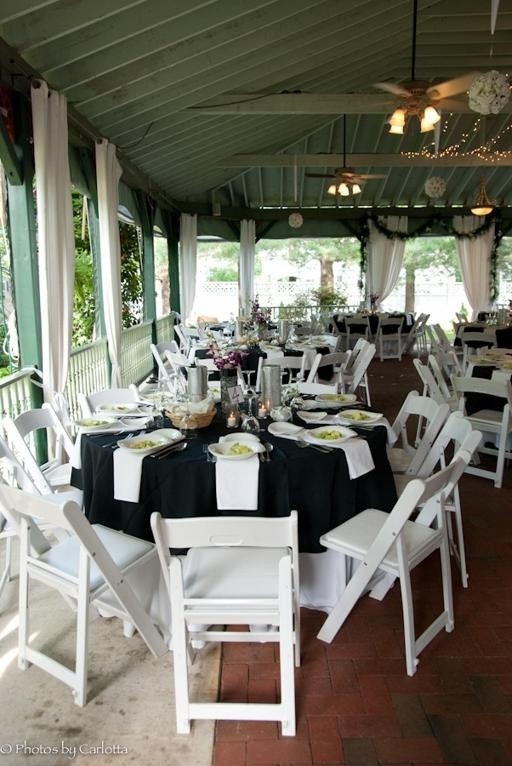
[68,428,122,471]
[490,367,512,384]
[483,325,509,334]
[261,347,286,358]
[187,346,210,365]
[113,434,187,503]
[292,347,318,357]
[305,415,399,451]
[406,314,414,327]
[455,323,479,338]
[223,326,233,335]
[214,452,259,511]
[463,361,497,383]
[337,314,345,322]
[274,427,376,481]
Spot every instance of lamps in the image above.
[469,169,497,217]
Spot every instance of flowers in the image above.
[423,176,448,199]
[467,70,510,117]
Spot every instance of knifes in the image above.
[151,442,184,457]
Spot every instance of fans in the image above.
[371,1,483,136]
[305,113,387,197]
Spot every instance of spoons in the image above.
[158,443,187,460]
[264,443,273,463]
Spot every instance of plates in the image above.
[315,393,357,404]
[96,403,138,414]
[74,417,115,430]
[305,426,358,444]
[117,434,169,453]
[207,439,264,462]
[336,410,383,424]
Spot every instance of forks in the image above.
[202,443,211,462]
[295,436,330,454]
[112,430,146,450]
[102,433,134,448]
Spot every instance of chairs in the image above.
[344,316,374,351]
[412,357,463,448]
[149,509,301,738]
[77,383,143,418]
[427,351,455,398]
[423,325,442,367]
[456,312,469,323]
[163,349,196,392]
[0,432,85,613]
[173,324,191,357]
[385,388,450,477]
[390,409,484,589]
[0,401,77,487]
[297,381,340,397]
[433,323,453,348]
[374,315,405,362]
[255,347,317,394]
[149,339,188,393]
[332,336,367,374]
[329,341,378,408]
[453,403,512,489]
[0,484,170,709]
[401,312,431,359]
[439,352,463,377]
[460,332,498,374]
[316,448,473,678]
[306,349,351,397]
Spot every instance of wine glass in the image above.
[241,394,261,434]
[146,388,164,433]
[241,370,257,401]
[177,392,199,440]
